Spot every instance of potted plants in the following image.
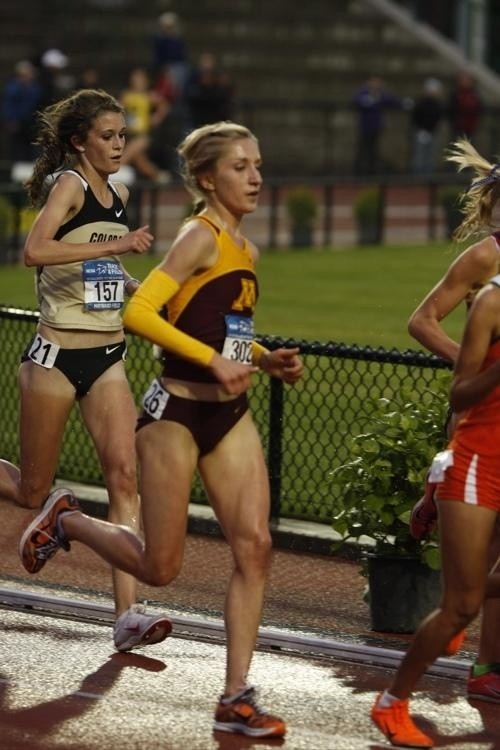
[325,397,443,636]
[285,187,468,251]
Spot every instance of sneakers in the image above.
[467,668,500,703]
[17,487,84,575]
[369,692,436,747]
[110,598,174,654]
[212,687,289,740]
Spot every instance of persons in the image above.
[408,136,500,657]
[2,87,174,652]
[445,69,484,167]
[346,74,407,178]
[400,76,451,174]
[17,118,290,741]
[1,12,236,185]
[369,269,500,749]
